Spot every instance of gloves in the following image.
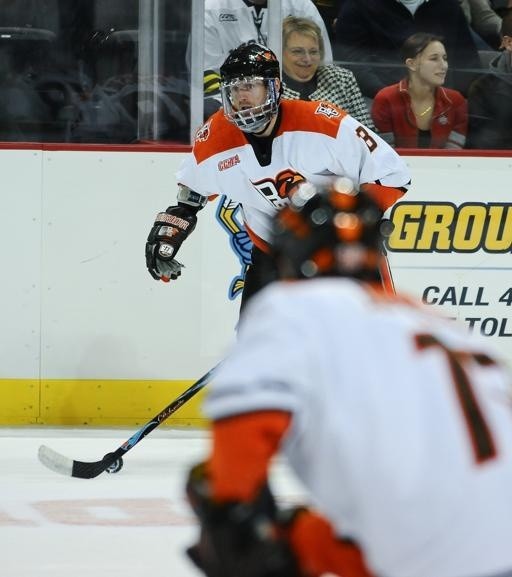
[146,207,197,282]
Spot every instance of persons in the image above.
[143,44,417,305]
[185,177,512,577]
[2,23,191,143]
[184,1,511,148]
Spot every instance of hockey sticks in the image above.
[39,351,223,480]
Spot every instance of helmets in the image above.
[273,182,385,284]
[220,44,279,133]
